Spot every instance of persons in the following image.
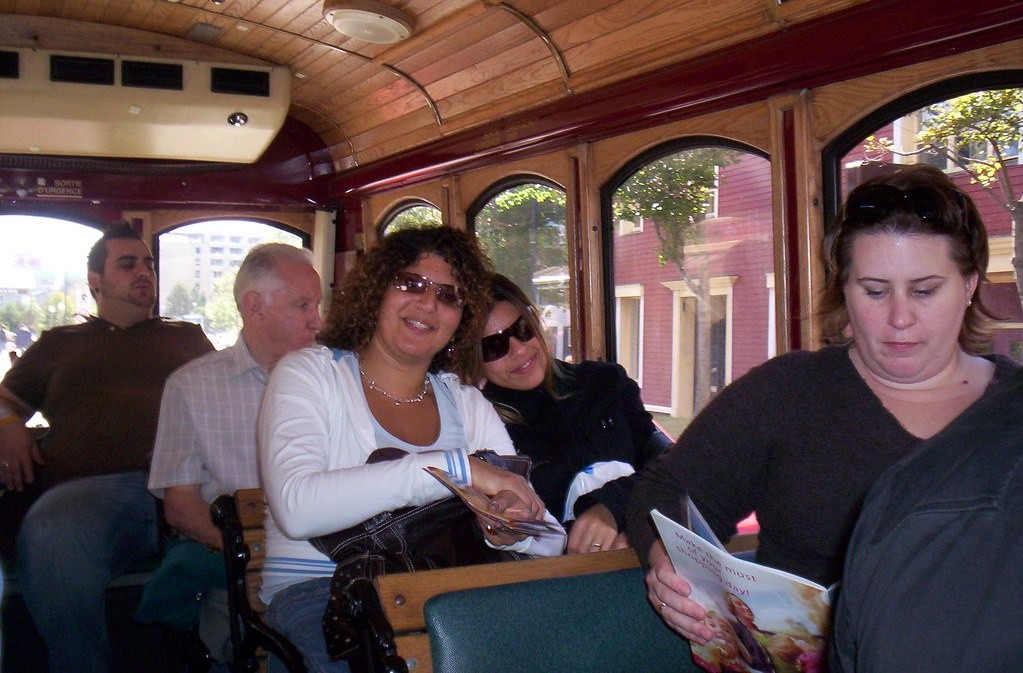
[703,591,775,673]
[135,244,323,673]
[0,224,218,673]
[258,226,545,673]
[466,271,669,555]
[623,164,1023,673]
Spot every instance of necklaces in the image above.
[359,365,430,406]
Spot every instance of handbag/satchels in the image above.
[305,446,532,673]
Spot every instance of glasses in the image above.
[471,304,540,363]
[390,270,469,310]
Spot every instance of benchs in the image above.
[370,533,756,673]
[211,485,307,672]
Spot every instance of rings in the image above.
[657,602,666,610]
[593,543,600,547]
[4,462,11,467]
[487,525,491,531]
